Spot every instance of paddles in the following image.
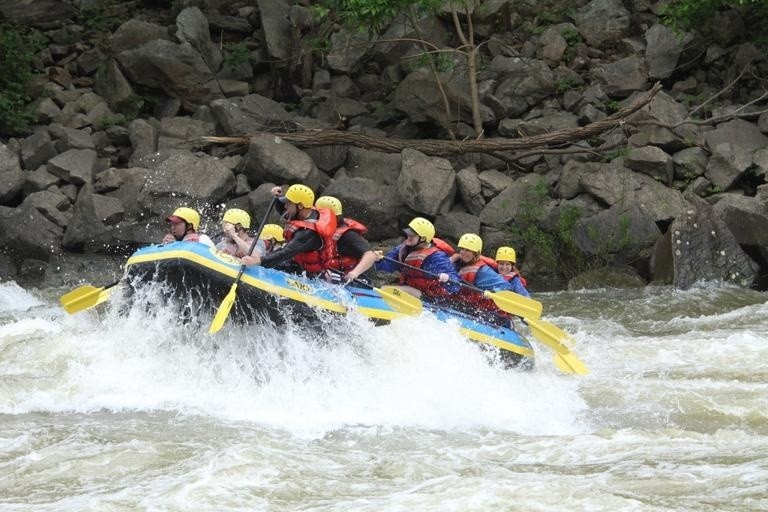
[327,267,422,317]
[517,316,588,375]
[379,255,543,320]
[60,280,120,314]
[209,189,281,332]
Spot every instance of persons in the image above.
[375,217,460,303]
[162,207,214,247]
[215,184,377,290]
[449,234,532,329]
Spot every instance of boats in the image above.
[123,242,533,372]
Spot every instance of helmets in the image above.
[259,225,286,242]
[457,233,483,255]
[495,246,517,264]
[401,216,436,243]
[165,206,201,232]
[314,195,343,216]
[221,208,251,229]
[277,183,315,209]
